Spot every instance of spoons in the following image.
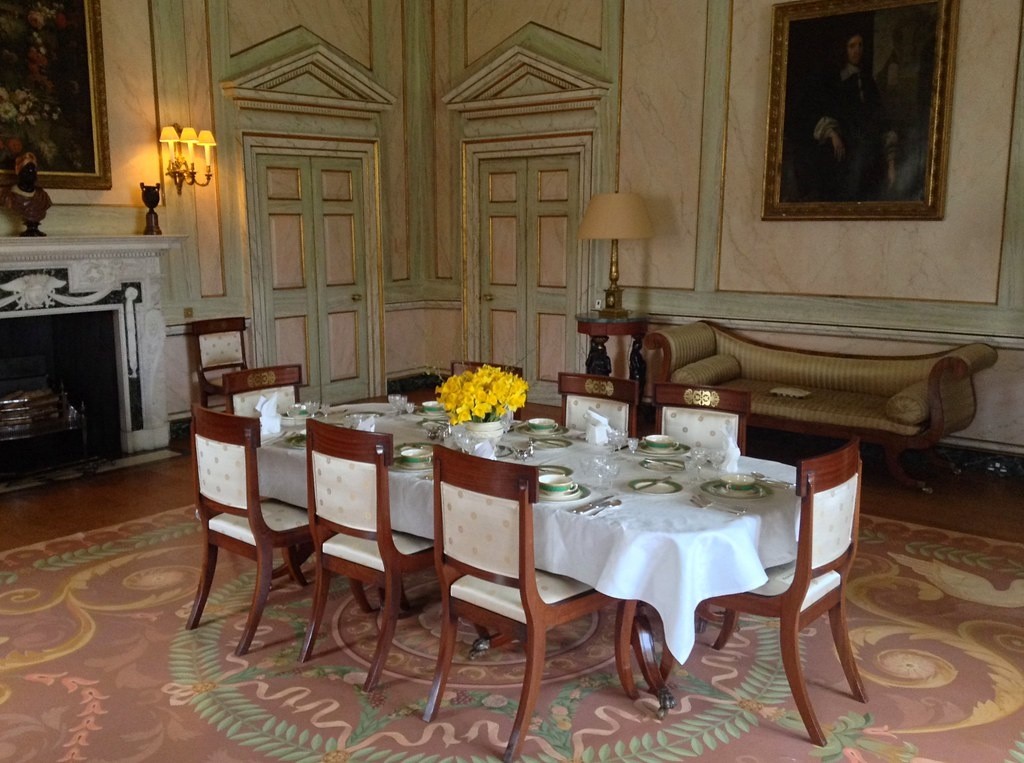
[752,471,794,486]
[591,499,621,517]
[690,496,747,515]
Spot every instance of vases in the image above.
[463,422,503,442]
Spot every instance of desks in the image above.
[575,312,653,387]
[256,401,801,721]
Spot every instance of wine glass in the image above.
[708,449,726,480]
[388,395,414,419]
[606,428,639,457]
[305,402,319,417]
[580,454,622,497]
[691,448,709,482]
[287,403,301,426]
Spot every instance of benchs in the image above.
[643,321,998,493]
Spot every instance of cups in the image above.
[583,407,607,427]
[455,431,479,456]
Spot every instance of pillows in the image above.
[885,378,929,428]
[669,353,737,388]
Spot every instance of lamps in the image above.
[574,191,656,319]
[158,126,218,196]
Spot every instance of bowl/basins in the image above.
[299,430,306,440]
[719,474,756,495]
[422,401,442,415]
[527,419,558,434]
[401,449,432,466]
[539,475,578,496]
[642,435,679,452]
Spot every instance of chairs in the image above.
[188,317,250,410]
[221,364,302,416]
[555,371,639,439]
[648,385,751,634]
[185,402,373,657]
[422,443,641,763]
[452,361,525,420]
[648,432,868,748]
[296,418,493,692]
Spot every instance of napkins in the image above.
[358,417,378,432]
[467,440,497,461]
[583,408,610,442]
[720,434,740,474]
[254,392,282,435]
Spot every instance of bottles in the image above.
[527,441,534,457]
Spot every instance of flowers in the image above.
[421,343,543,427]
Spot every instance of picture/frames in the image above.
[760,0,960,223]
[0,0,111,190]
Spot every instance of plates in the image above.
[637,442,691,457]
[639,460,685,473]
[514,424,569,436]
[394,442,433,454]
[283,436,307,448]
[700,481,773,501]
[458,445,513,458]
[392,457,434,472]
[539,484,591,503]
[343,411,385,421]
[536,465,574,478]
[528,439,573,449]
[279,411,324,419]
[628,479,682,495]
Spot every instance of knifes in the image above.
[645,459,682,469]
[570,495,615,513]
[634,475,671,491]
